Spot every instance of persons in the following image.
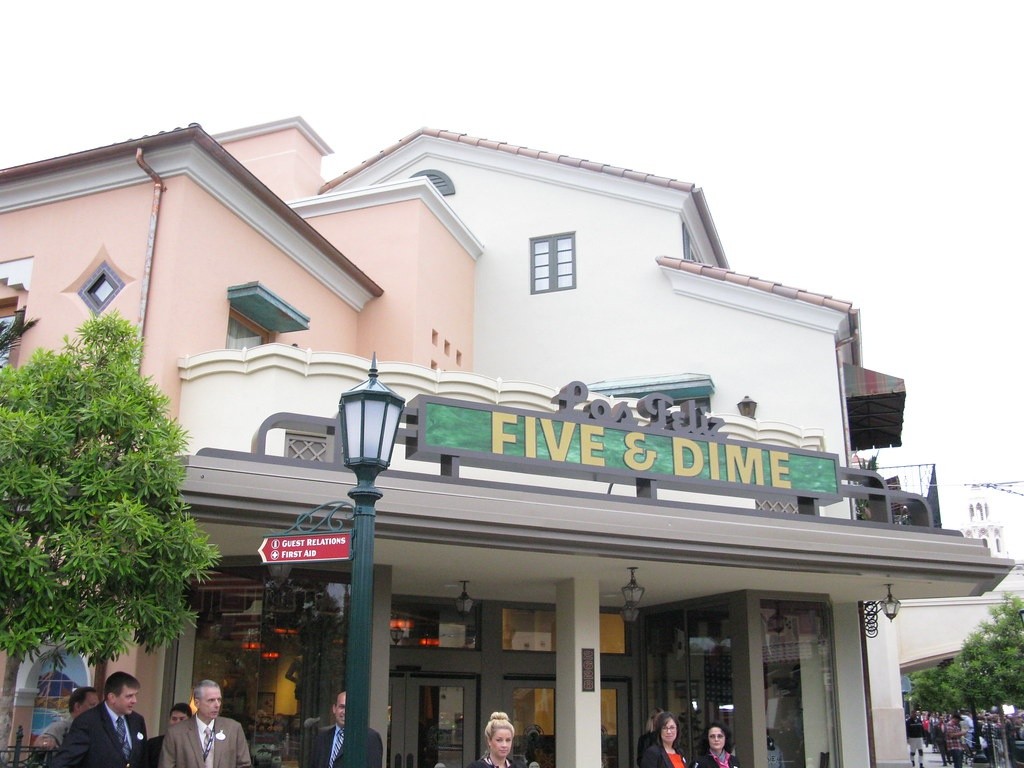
[147,703,193,768]
[766,730,786,768]
[34,686,100,768]
[285,650,303,713]
[778,717,804,768]
[686,721,741,768]
[906,708,1024,768]
[52,671,147,768]
[636,707,665,768]
[641,711,689,768]
[158,679,252,768]
[466,711,528,768]
[308,690,383,768]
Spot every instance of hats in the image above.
[953,713,965,721]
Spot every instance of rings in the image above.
[43,742,47,744]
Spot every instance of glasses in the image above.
[661,726,677,732]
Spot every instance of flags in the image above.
[37,672,51,686]
[41,683,50,697]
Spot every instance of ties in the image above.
[117,716,131,762]
[328,730,345,768]
[204,727,212,758]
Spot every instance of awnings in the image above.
[842,360,908,451]
